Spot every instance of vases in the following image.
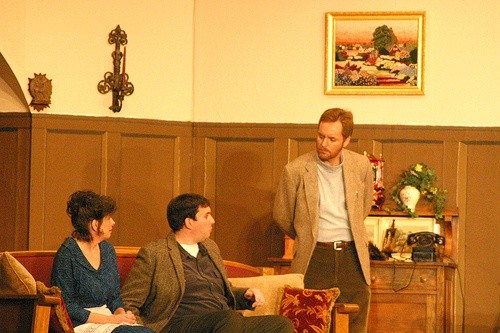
[400,185,421,212]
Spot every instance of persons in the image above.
[274,108,373,333]
[50,191,155,333]
[119,194,295,333]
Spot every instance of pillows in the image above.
[0,250,37,295]
[279,284,340,333]
[227,274,304,316]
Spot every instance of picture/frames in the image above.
[324,10,425,95]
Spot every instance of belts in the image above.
[316,240,354,250]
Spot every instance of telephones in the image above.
[407,232,445,262]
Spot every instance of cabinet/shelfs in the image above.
[266,207,459,333]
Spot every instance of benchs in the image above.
[0,246,360,333]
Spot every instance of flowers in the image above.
[390,163,446,219]
